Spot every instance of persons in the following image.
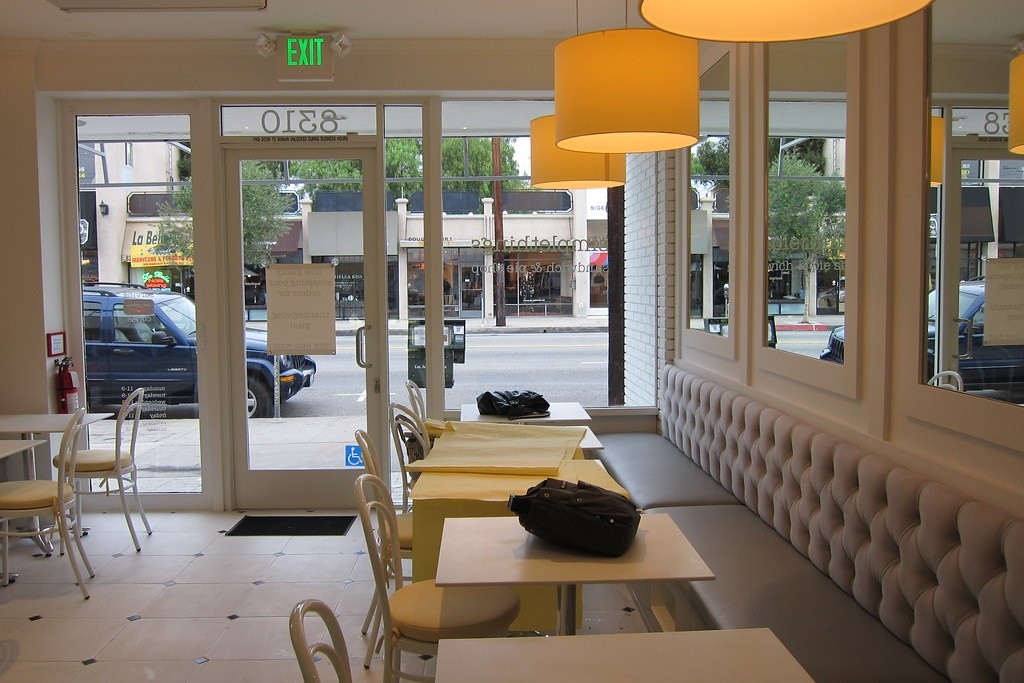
[520,265,534,312]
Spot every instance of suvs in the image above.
[82,281,316,420]
[820,276,1024,389]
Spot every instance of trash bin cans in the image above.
[703,318,728,335]
[767,316,777,347]
[408,319,454,389]
[445,320,465,364]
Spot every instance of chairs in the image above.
[287,380,521,683]
[45,388,153,558]
[0,407,96,599]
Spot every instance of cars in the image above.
[819,278,846,304]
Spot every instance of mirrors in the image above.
[923,1,1024,408]
[683,48,730,339]
[763,35,845,367]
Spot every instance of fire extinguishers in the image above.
[56,356,80,414]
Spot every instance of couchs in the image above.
[584,363,1024,683]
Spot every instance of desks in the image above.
[432,400,815,683]
[0,412,115,553]
[0,438,48,584]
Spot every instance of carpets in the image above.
[225,515,357,536]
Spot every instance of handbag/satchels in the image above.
[508,478,642,558]
[476,389,551,420]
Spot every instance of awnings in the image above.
[1000,187,1024,244]
[929,186,995,244]
[244,219,302,258]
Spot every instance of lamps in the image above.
[930,116,945,187]
[638,0,938,43]
[529,0,626,189]
[99,200,108,217]
[1007,44,1024,157]
[553,0,700,155]
[254,29,352,82]
